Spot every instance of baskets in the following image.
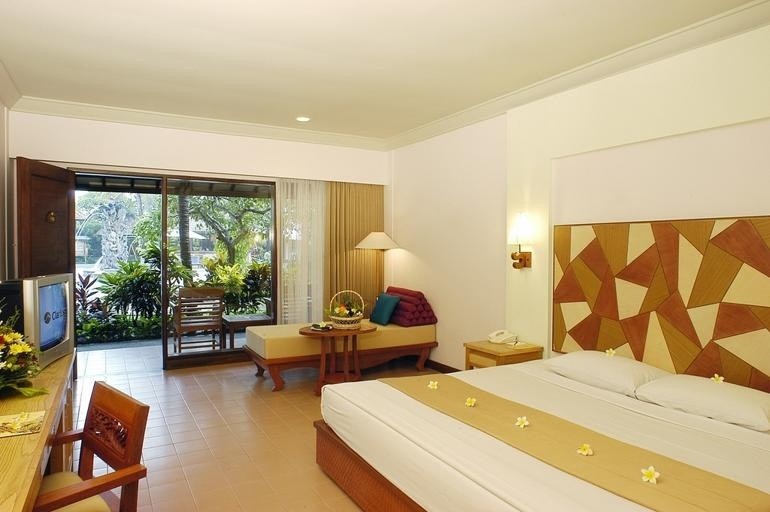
[327,289,366,331]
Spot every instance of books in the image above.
[0,410,47,439]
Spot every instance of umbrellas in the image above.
[166,228,209,240]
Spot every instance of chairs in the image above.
[83,256,103,275]
[33,379,150,512]
[169,288,224,353]
[264,297,271,315]
[191,256,201,268]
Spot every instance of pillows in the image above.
[636,374,770,433]
[370,286,438,327]
[544,351,673,399]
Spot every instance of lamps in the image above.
[508,216,532,268]
[356,232,398,249]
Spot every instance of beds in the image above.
[313,359,770,512]
[242,318,438,392]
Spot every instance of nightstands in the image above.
[463,341,543,370]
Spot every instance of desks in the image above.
[299,325,376,396]
[0,349,76,512]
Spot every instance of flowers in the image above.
[577,443,593,457]
[465,398,476,407]
[0,297,50,397]
[641,466,659,484]
[428,381,438,389]
[516,416,529,428]
[711,374,724,384]
[319,321,326,327]
[323,294,368,317]
[606,348,615,356]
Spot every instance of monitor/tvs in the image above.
[0,271,76,376]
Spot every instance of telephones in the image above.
[488,330,518,344]
